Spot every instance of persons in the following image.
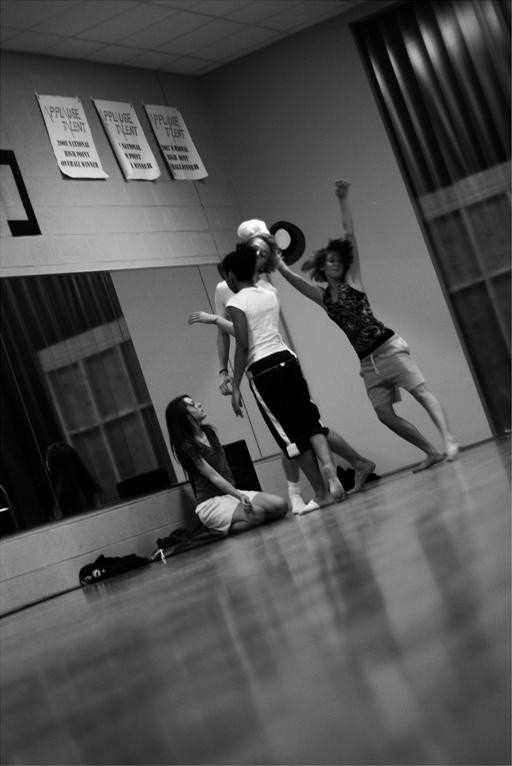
[46,440,108,517]
[165,393,289,558]
[237,177,461,474]
[216,248,347,515]
[215,232,376,508]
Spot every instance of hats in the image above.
[237,219,271,243]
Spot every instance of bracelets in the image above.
[218,368,229,375]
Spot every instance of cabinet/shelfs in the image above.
[37,316,179,506]
[419,161,512,440]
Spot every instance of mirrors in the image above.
[0,263,282,542]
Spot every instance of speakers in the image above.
[223,439,263,492]
[115,464,174,497]
